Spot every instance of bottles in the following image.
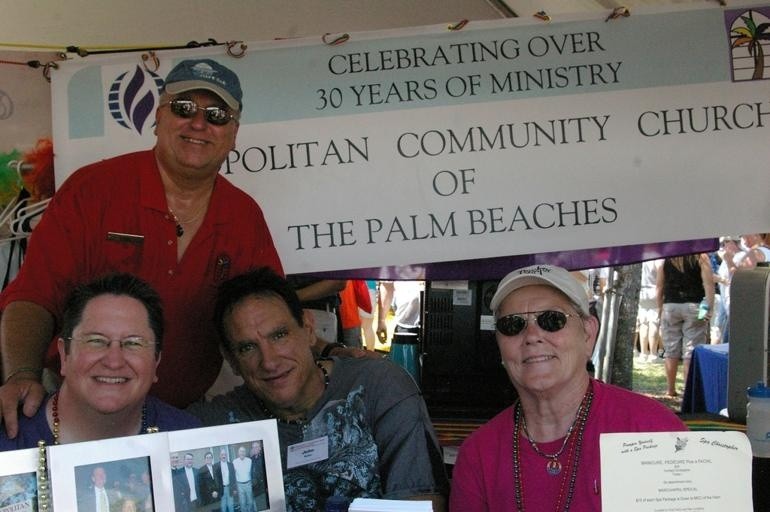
[390,331,420,383]
[745,381,770,460]
[697,298,710,320]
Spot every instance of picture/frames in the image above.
[46,432,176,512]
[170,419,287,511]
[0,447,53,510]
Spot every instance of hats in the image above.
[163,58,243,112]
[490,265,590,320]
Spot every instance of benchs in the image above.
[431,422,749,490]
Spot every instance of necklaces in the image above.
[258,358,330,425]
[164,178,213,237]
[52,388,147,439]
[513,393,592,512]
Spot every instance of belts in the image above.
[302,301,337,315]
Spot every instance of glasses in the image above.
[496,310,567,337]
[161,96,238,127]
[67,333,157,355]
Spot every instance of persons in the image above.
[449,264,690,512]
[0,273,205,452]
[635,233,770,404]
[184,268,450,512]
[0,57,383,439]
[169,440,269,512]
[79,466,150,512]
[294,275,423,358]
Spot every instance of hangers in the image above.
[1,157,60,243]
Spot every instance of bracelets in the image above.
[2,366,35,382]
